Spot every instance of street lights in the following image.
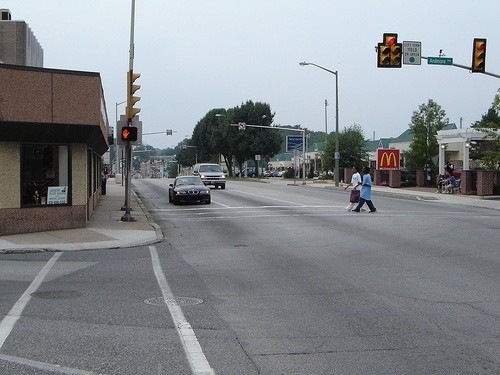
[299,62,339,187]
[324,99,328,135]
[260,115,266,134]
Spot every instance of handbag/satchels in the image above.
[350,190,360,203]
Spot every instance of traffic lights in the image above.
[377,33,402,68]
[472,38,487,73]
[121,126,138,141]
[126,72,141,121]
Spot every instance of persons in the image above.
[352,167,376,213]
[437,171,456,193]
[345,167,366,211]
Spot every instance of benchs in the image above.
[435,180,461,194]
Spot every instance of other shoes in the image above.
[369,208,376,213]
[352,208,360,212]
[361,208,366,211]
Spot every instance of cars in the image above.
[168,176,211,206]
[313,173,320,177]
[234,168,285,178]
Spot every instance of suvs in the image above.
[192,163,227,189]
